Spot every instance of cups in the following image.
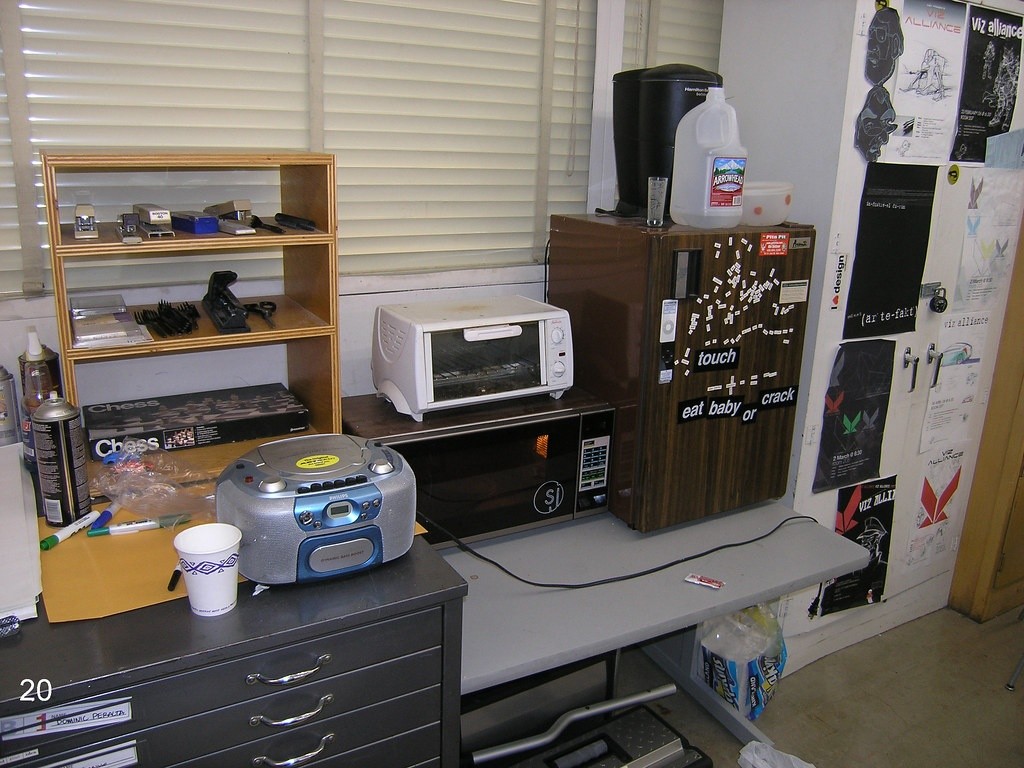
[647,175,669,228]
[172,523,243,617]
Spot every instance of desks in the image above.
[437,498,871,747]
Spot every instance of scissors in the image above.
[246,300,278,329]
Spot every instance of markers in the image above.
[40,509,101,551]
[90,492,124,529]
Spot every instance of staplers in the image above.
[116,213,144,243]
[74,204,99,239]
[203,198,257,236]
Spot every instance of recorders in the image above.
[215,433,416,584]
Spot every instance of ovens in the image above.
[370,294,575,422]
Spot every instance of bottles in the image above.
[0,365,23,448]
[19,344,63,474]
[670,86,749,228]
[29,391,91,529]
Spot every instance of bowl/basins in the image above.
[738,180,794,227]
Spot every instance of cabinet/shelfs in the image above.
[0,531,468,768]
[40,150,344,499]
[945,214,1022,623]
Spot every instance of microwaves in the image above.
[340,392,616,552]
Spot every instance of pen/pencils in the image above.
[88,511,193,537]
[167,559,183,591]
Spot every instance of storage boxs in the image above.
[82,383,309,461]
